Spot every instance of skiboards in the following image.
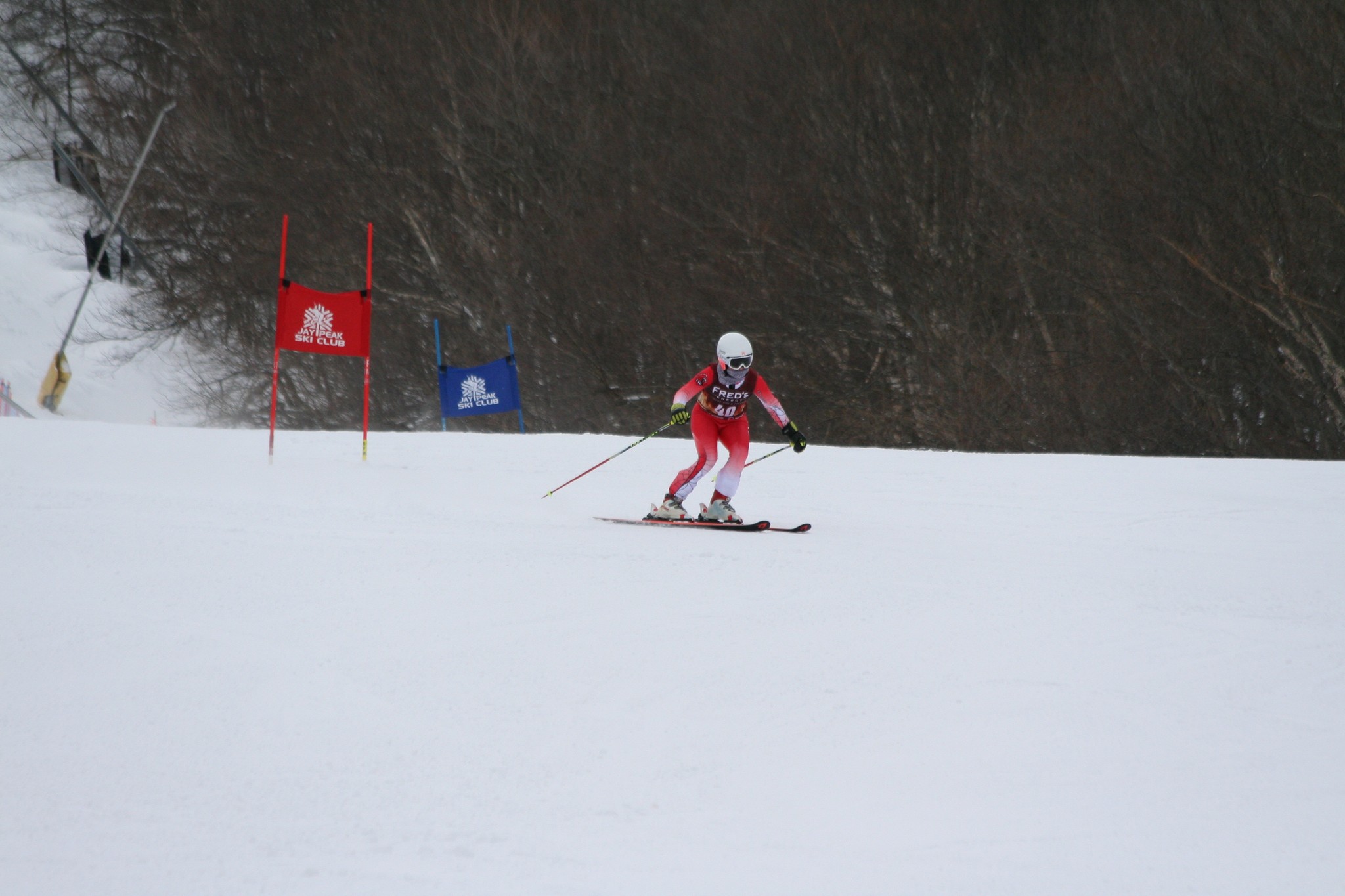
[594,515,813,534]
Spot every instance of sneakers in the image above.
[657,493,695,522]
[705,491,743,523]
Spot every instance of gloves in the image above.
[782,421,807,453]
[670,403,690,424]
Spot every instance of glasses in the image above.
[728,355,752,370]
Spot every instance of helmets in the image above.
[716,333,753,371]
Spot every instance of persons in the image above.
[656,333,807,523]
